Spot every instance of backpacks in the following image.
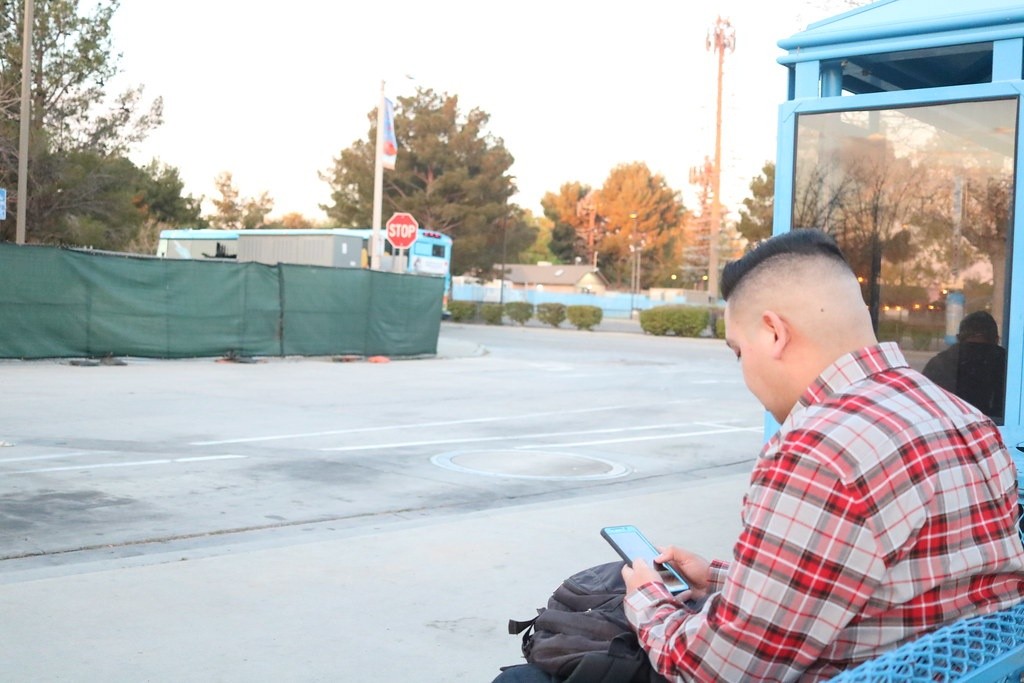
[508,560,664,683]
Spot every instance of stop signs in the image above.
[386,212,418,249]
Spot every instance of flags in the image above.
[382,97,398,170]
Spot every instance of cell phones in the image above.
[601,524,690,598]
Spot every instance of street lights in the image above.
[697,15,736,338]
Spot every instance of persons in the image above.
[917,310,1006,421]
[491,226,1024,683]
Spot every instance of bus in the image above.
[156,229,453,320]
